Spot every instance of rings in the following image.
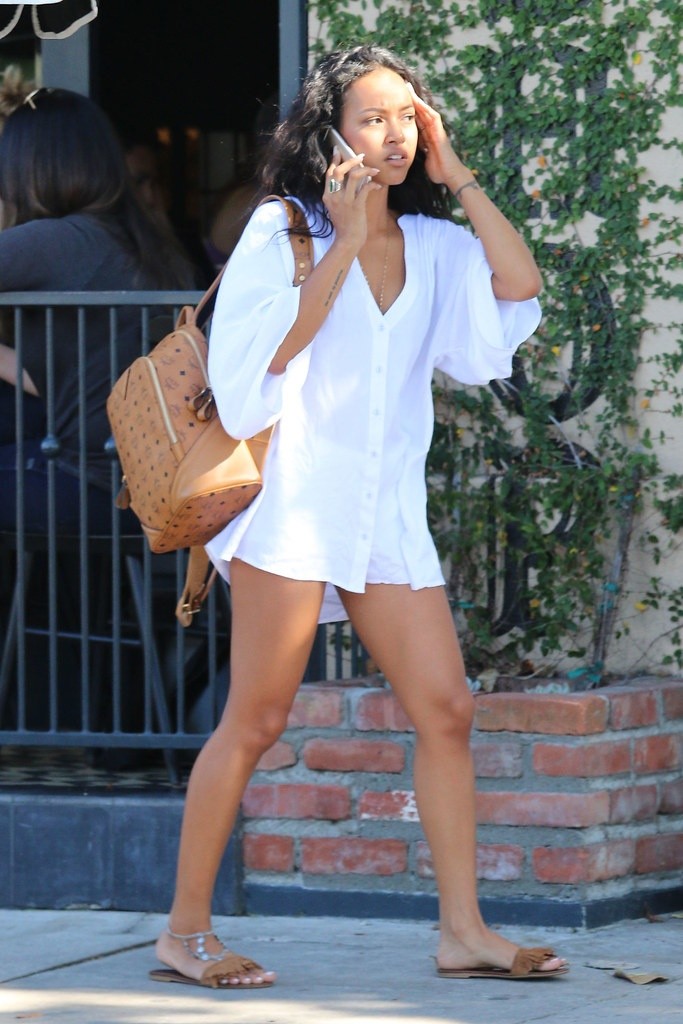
[329,179,346,194]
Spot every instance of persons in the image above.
[1,86,191,537]
[150,45,571,992]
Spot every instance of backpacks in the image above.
[106,194,313,626]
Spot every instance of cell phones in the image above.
[321,125,373,199]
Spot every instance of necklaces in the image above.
[378,207,391,309]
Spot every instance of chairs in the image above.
[0,247,228,790]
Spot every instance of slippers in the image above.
[438,946,570,980]
[149,954,275,989]
